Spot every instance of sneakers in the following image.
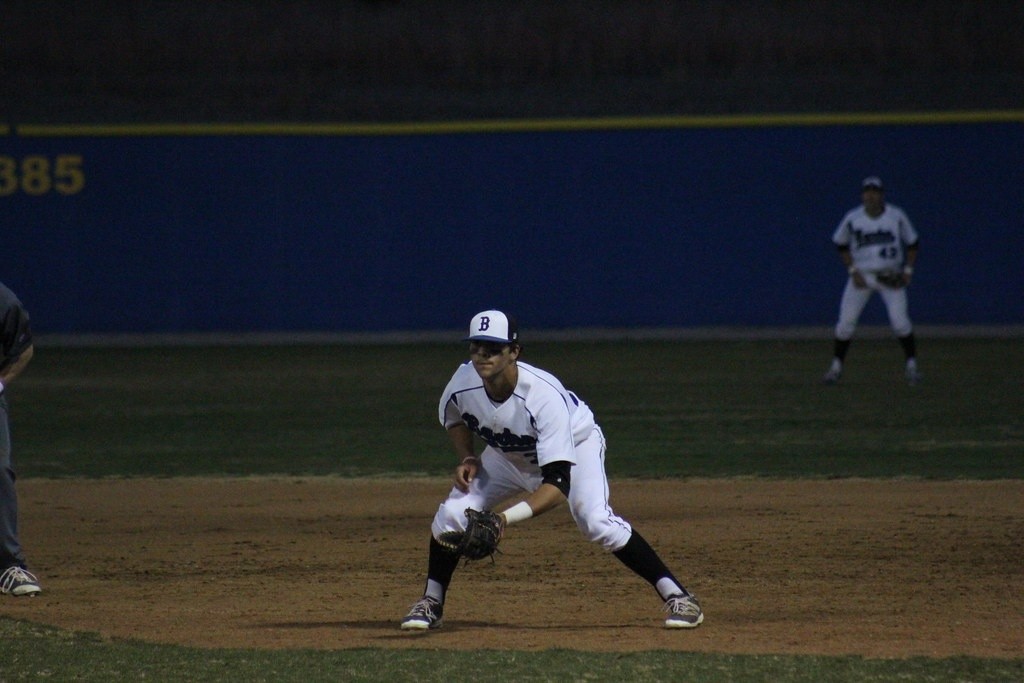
[0,565,42,596]
[662,592,704,628]
[401,596,443,629]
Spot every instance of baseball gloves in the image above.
[872,266,912,287]
[436,506,506,561]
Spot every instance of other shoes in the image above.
[815,370,842,387]
[906,368,920,386]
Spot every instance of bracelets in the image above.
[848,266,857,274]
[502,500,532,528]
[904,266,914,274]
[463,456,475,463]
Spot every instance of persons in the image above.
[0,281,42,595]
[402,310,703,630]
[819,177,921,387]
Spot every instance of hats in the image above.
[461,308,521,343]
[860,176,884,193]
